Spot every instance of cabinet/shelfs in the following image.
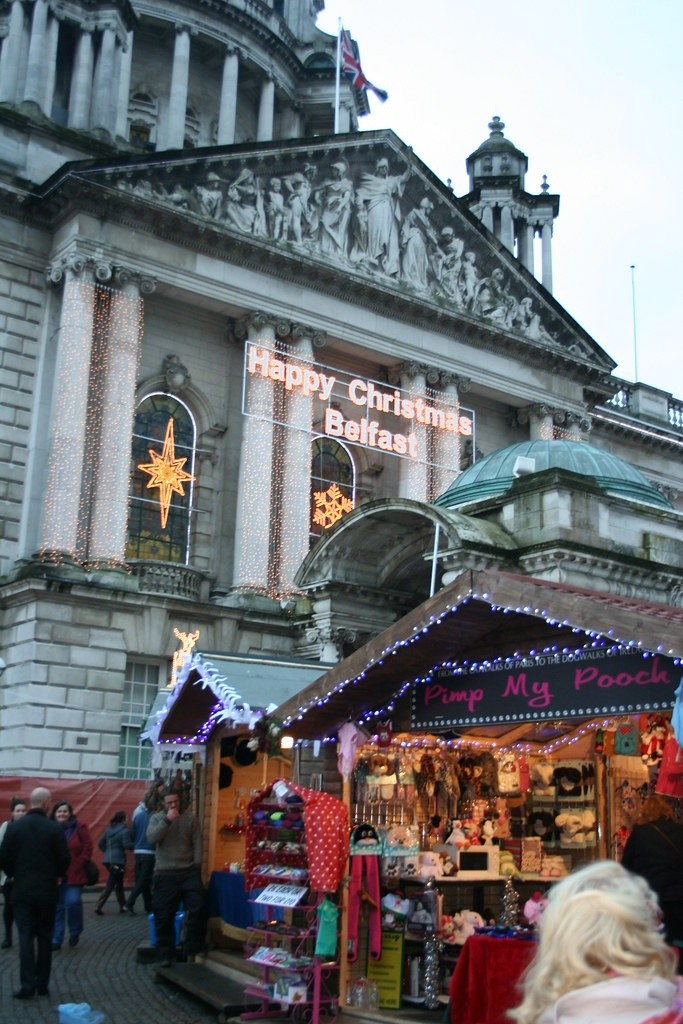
[241,872,345,1024]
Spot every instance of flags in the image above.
[341,26,388,102]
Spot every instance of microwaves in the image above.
[433,843,500,876]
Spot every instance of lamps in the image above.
[512,457,535,478]
[280,736,293,748]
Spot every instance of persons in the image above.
[94,811,131,915]
[620,796,683,939]
[506,860,683,1024]
[146,788,206,948]
[123,780,164,915]
[154,146,533,328]
[0,787,71,1000]
[49,801,94,952]
[0,796,27,947]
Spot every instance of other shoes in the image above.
[123,904,137,915]
[93,908,103,915]
[69,934,80,947]
[0,940,12,949]
[51,943,61,951]
[162,955,174,969]
[13,991,35,999]
[37,984,49,995]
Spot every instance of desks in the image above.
[440,935,539,1024]
[379,875,563,1006]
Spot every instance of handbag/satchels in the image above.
[84,859,100,885]
